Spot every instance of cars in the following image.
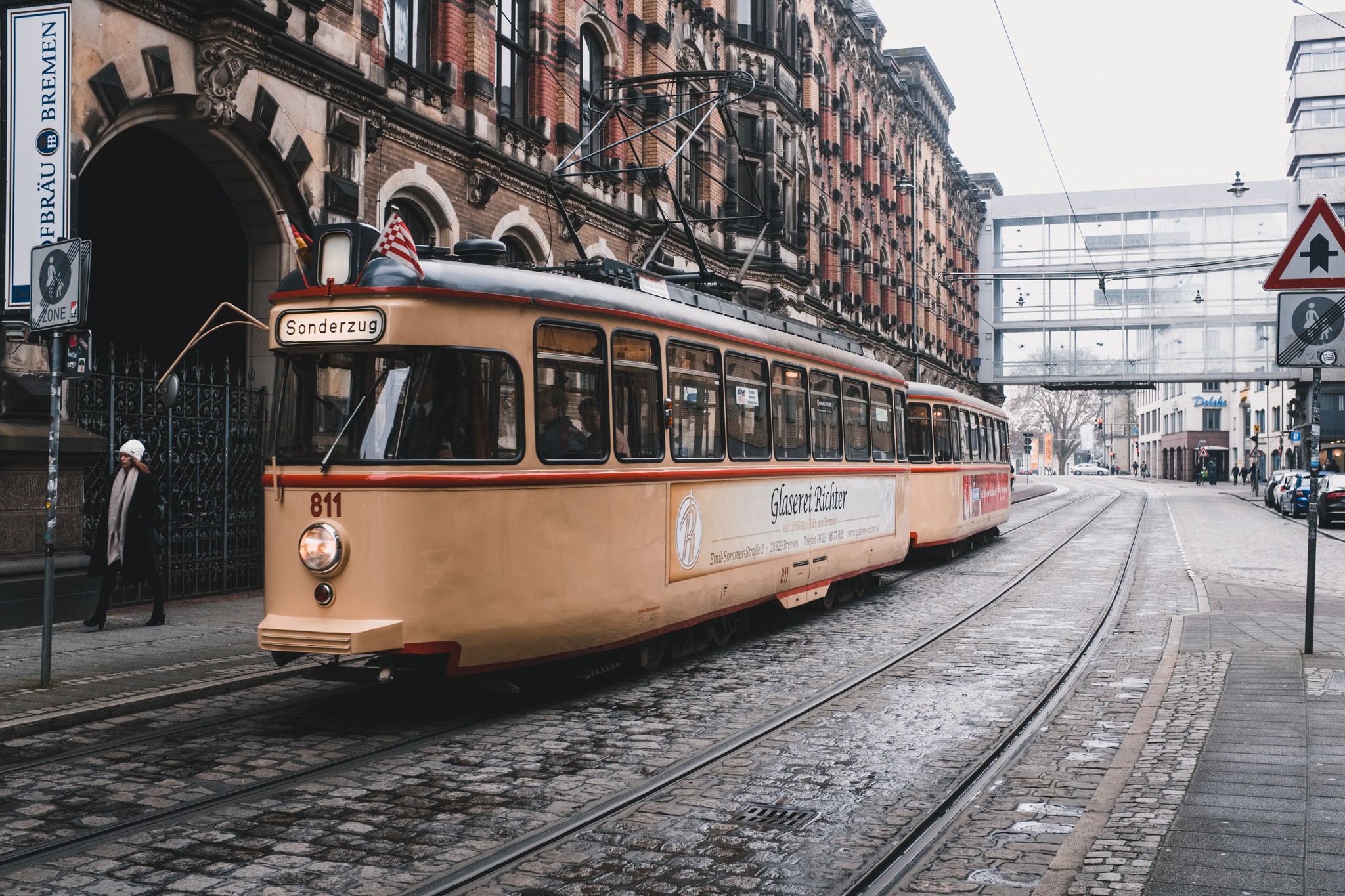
[1306,472,1345,529]
[1070,463,1109,475]
[1017,468,1033,475]
[1264,468,1336,519]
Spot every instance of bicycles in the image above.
[1133,467,1139,477]
[1249,472,1259,492]
[1140,468,1147,479]
[1240,472,1250,485]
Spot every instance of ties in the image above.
[406,405,426,456]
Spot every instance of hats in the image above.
[119,439,145,461]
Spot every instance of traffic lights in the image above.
[1113,453,1116,459]
[1098,419,1103,429]
[1137,449,1139,456]
[1110,452,1113,456]
[1122,426,1127,435]
[1136,441,1138,448]
[1086,453,1091,460]
[1094,420,1097,427]
[1250,436,1258,442]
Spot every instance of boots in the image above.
[145,603,165,626]
[84,605,108,630]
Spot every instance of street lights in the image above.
[1067,463,1069,475]
[1041,466,1042,475]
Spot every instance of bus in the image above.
[155,70,1009,682]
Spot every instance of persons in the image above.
[390,364,473,459]
[1327,459,1340,472]
[1322,459,1330,471]
[1231,463,1240,485]
[1011,462,1014,491]
[83,439,166,630]
[1048,463,1052,475]
[1111,466,1115,475]
[577,398,631,458]
[537,387,588,459]
[1194,463,1202,485]
[1105,464,1109,475]
[1239,465,1248,485]
[1248,463,1259,485]
[1140,461,1146,477]
[1115,466,1121,474]
[1133,462,1138,476]
[1098,461,1100,467]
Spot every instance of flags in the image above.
[374,211,425,287]
[283,214,314,269]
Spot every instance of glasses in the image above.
[538,401,555,410]
[579,412,600,423]
